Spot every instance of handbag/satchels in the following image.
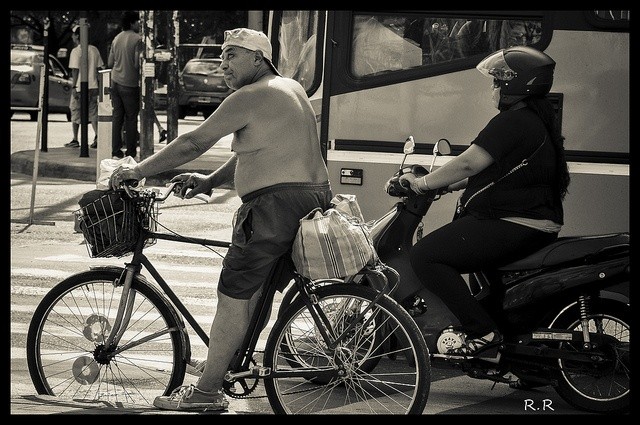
[290,193,379,281]
[451,128,548,222]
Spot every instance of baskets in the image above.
[75,191,160,258]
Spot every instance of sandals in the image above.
[444,329,501,357]
[509,378,529,389]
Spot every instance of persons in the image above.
[109,27,333,412]
[399,45,564,356]
[136,93,168,147]
[64,21,104,148]
[107,11,143,160]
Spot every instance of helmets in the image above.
[475,46,557,111]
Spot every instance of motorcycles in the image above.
[279,135,631,411]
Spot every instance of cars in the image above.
[11,45,78,120]
[177,58,232,120]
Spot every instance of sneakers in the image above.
[153,384,230,410]
[64,142,78,147]
[185,358,237,393]
[90,139,97,148]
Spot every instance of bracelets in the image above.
[423,176,432,191]
[412,178,426,196]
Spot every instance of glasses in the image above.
[493,78,500,88]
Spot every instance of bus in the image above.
[268,9,628,245]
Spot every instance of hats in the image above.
[66,19,90,34]
[221,28,283,78]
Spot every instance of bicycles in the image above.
[26,178,432,416]
